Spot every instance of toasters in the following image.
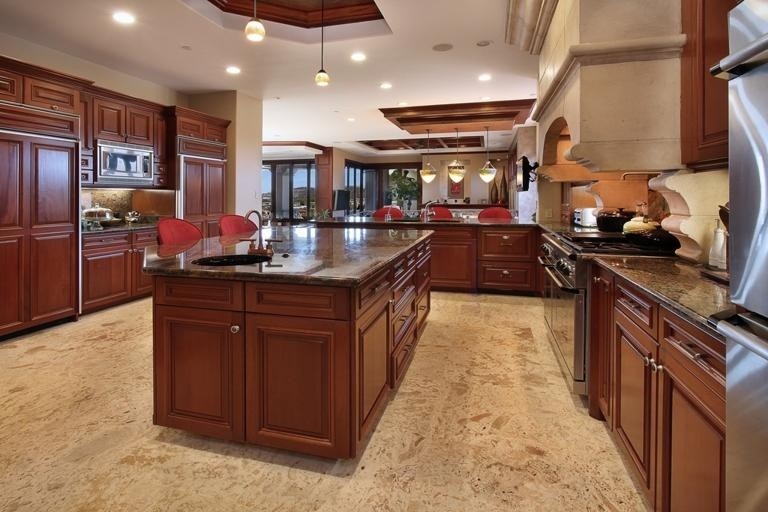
[572,207,604,227]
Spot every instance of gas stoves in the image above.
[540,231,678,289]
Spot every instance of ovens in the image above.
[537,255,589,397]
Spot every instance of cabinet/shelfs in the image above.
[585,254,727,512]
[680,0,731,170]
[142,223,432,462]
[0,129,78,340]
[167,105,234,145]
[171,135,227,241]
[80,139,171,188]
[79,226,133,317]
[477,224,542,298]
[92,84,156,148]
[135,225,160,299]
[422,223,478,294]
[0,68,25,107]
[23,75,82,117]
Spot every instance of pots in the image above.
[597,207,631,232]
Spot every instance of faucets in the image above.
[424,200,437,222]
[244,209,263,250]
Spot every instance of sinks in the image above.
[191,254,272,266]
[429,220,460,222]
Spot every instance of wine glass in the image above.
[125,212,136,230]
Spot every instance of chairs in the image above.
[156,218,203,244]
[421,206,452,220]
[219,213,258,237]
[478,207,511,219]
[375,207,403,218]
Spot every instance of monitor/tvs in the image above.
[516,155,530,193]
[333,189,350,210]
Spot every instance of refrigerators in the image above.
[709,0,768,512]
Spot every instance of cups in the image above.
[384,214,393,221]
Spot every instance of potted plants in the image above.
[387,169,421,210]
[318,209,329,220]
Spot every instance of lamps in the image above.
[315,0,331,88]
[420,129,436,184]
[244,1,264,42]
[447,127,467,184]
[479,125,497,184]
